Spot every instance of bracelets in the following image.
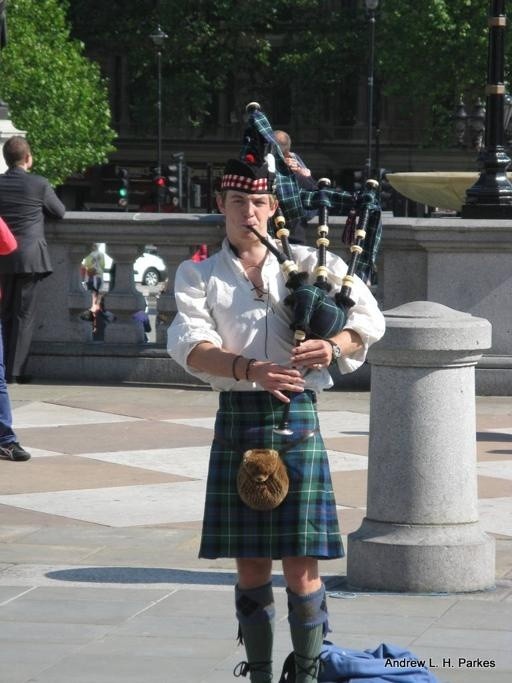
[233,355,257,382]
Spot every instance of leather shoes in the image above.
[1,443,29,460]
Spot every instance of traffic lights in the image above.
[168,165,178,194]
[117,166,129,209]
[153,176,168,199]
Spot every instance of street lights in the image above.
[150,22,170,213]
[364,0,379,179]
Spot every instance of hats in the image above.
[221,158,277,194]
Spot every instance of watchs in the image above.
[328,339,340,369]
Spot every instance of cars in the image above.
[100,246,166,287]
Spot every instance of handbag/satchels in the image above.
[238,448,290,510]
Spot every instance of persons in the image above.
[167,160,386,683]
[270,130,312,244]
[0,136,66,461]
